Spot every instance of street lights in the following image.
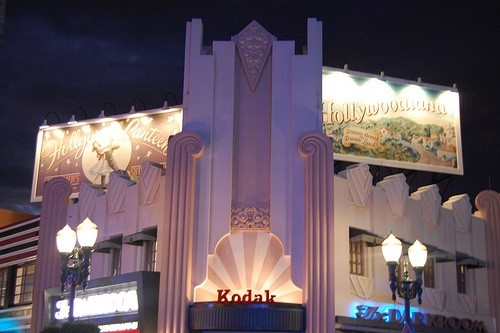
[381,230,429,333]
[55,216,99,323]
